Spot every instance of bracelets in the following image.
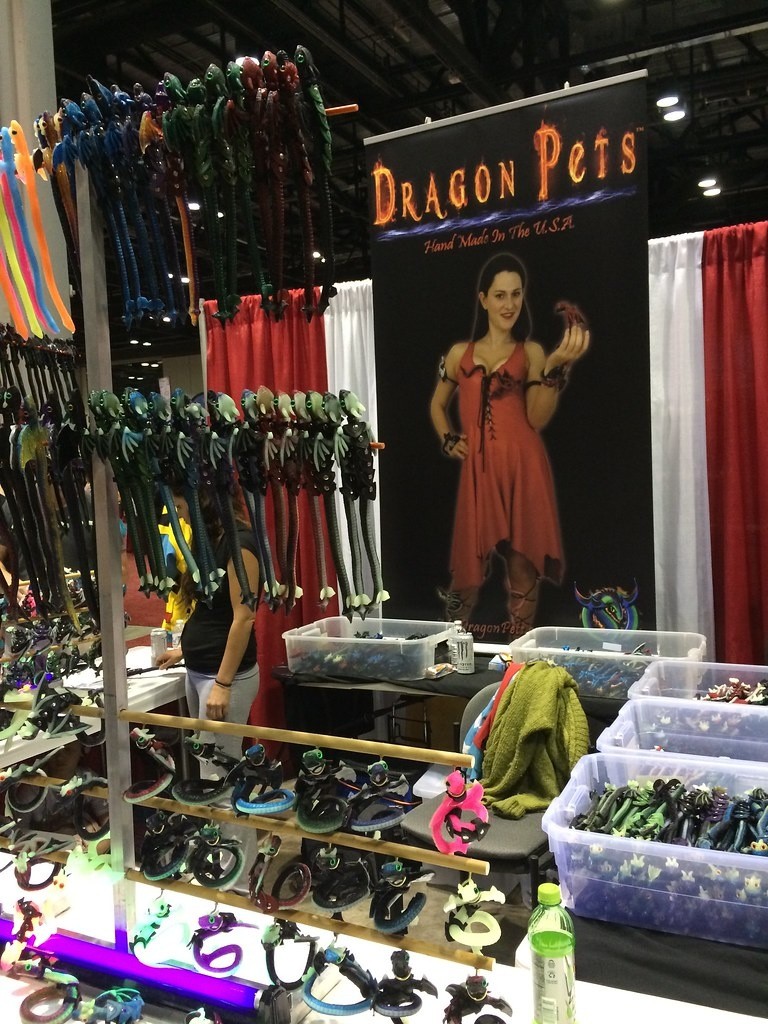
[541,365,567,390]
[442,433,453,455]
[215,679,232,687]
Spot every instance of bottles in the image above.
[451,621,466,670]
[172,620,184,665]
[528,883,576,1024]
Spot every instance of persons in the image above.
[157,472,268,896]
[428,253,591,641]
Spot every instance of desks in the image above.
[0,644,187,781]
[271,653,628,753]
[4,846,768,1024]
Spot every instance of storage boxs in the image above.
[597,700,768,799]
[627,661,768,743]
[509,626,708,700]
[541,753,768,949]
[281,617,454,681]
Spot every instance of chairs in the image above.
[401,680,589,914]
